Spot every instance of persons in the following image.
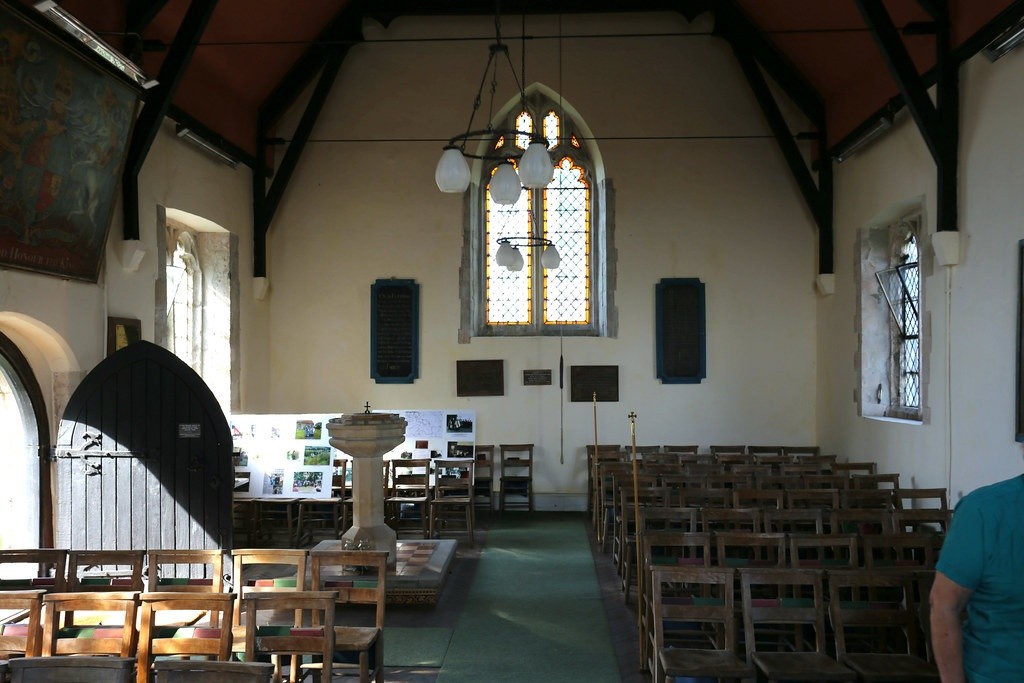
[927,474,1024,683]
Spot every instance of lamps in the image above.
[495,0,560,272]
[435,0,553,199]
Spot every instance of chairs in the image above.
[0,444,953,683]
